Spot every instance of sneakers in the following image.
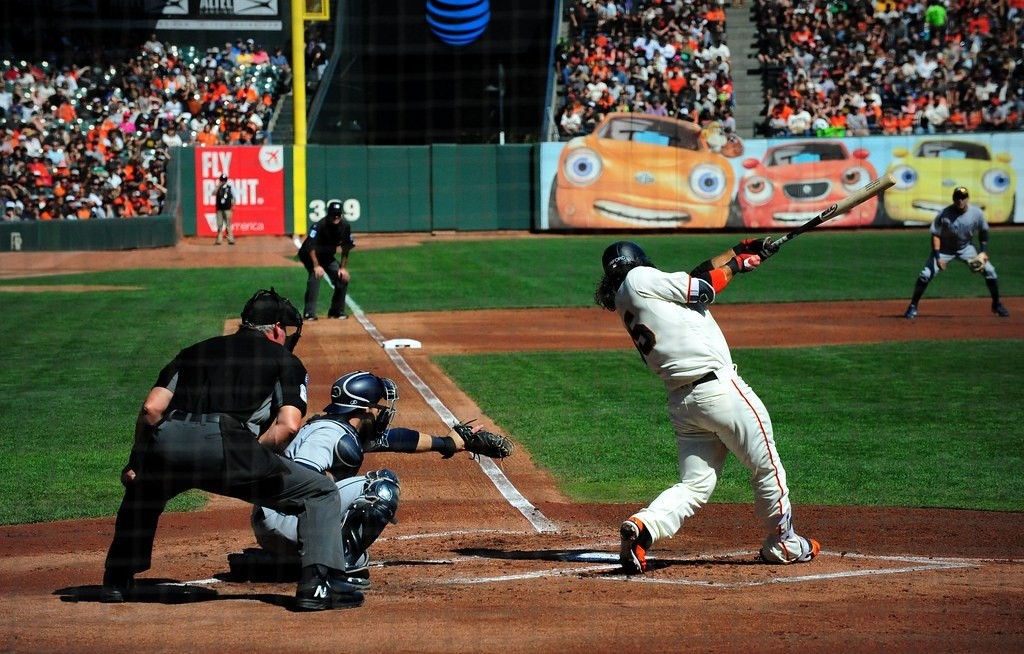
[228,553,249,575]
[905,304,917,318]
[992,302,1009,316]
[104,570,143,604]
[761,537,820,564]
[621,518,646,573]
[328,310,348,320]
[296,577,364,609]
[304,312,318,320]
[345,576,371,589]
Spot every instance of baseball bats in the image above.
[771,173,900,247]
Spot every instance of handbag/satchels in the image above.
[219,412,292,488]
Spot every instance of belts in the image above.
[162,411,221,423]
[681,372,718,389]
[253,508,267,525]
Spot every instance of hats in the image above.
[953,187,969,195]
[329,202,343,212]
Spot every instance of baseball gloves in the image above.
[967,252,988,273]
[453,423,515,459]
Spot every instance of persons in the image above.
[212,175,236,245]
[552,0,736,140]
[902,187,1008,319]
[103,286,364,611]
[305,29,329,88]
[731,0,746,9]
[594,237,819,573]
[298,202,351,320]
[753,0,1024,133]
[0,33,294,220]
[228,369,515,591]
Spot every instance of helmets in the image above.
[603,241,646,273]
[241,287,303,328]
[322,371,399,446]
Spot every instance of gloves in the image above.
[740,237,772,254]
[736,253,761,272]
